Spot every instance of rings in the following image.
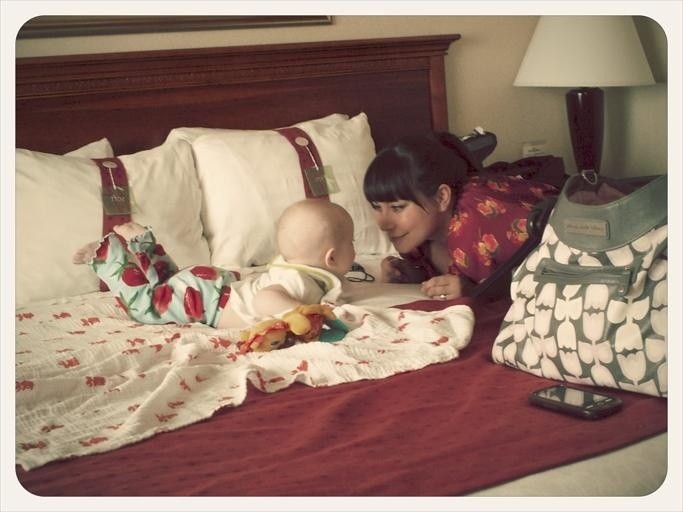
[439,294,447,300]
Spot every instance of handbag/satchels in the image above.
[470,167,667,401]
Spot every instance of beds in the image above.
[14,35,668,502]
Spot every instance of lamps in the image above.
[511,13,658,180]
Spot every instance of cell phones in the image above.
[529,384,623,420]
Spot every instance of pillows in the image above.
[174,109,408,268]
[15,138,217,316]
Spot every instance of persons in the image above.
[71,196,355,331]
[362,130,560,302]
